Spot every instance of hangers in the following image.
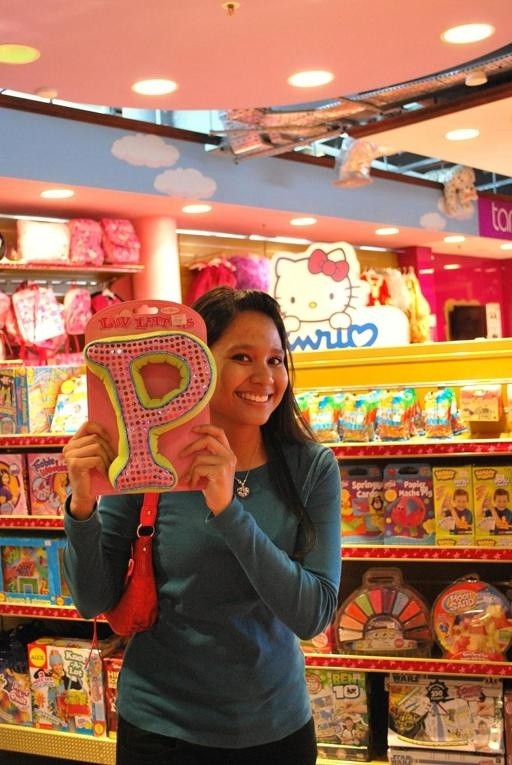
[359,265,416,278]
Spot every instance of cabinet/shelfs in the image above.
[0,335,512,765]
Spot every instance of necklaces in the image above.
[234,431,264,501]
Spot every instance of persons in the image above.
[58,286,343,764]
[33,650,83,692]
[476,488,512,534]
[0,467,13,506]
[439,488,474,535]
